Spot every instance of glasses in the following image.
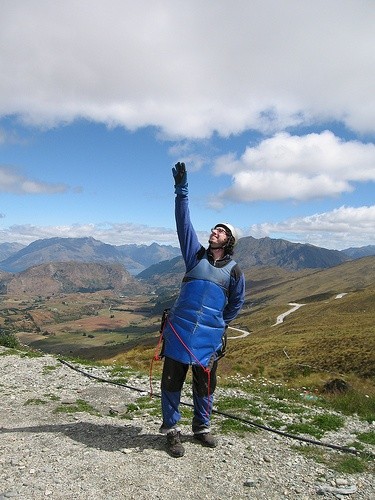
[211,228,229,237]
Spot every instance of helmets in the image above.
[213,223,237,247]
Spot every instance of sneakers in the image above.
[167,431,185,457]
[194,432,216,448]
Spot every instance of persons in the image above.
[158,162,245,459]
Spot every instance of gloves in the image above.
[172,162,188,197]
